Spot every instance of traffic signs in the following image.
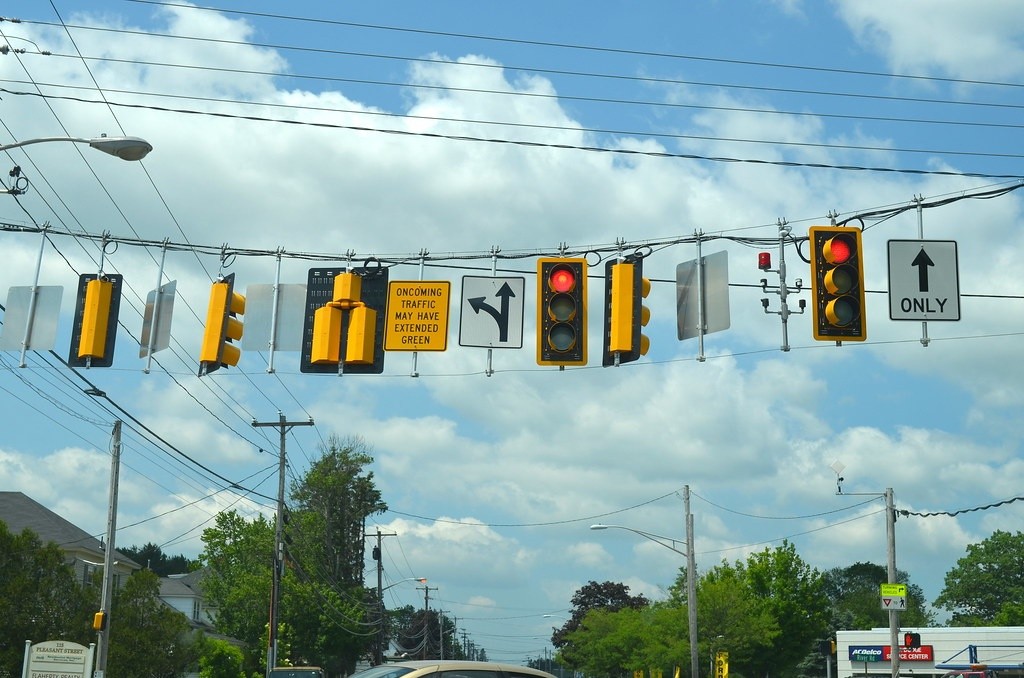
[886,237,962,323]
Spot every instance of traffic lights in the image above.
[905,632,920,650]
[198,271,250,372]
[809,225,870,345]
[93,612,107,633]
[540,255,593,370]
[600,250,654,368]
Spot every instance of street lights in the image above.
[375,576,427,665]
[591,522,698,678]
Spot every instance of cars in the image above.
[348,659,555,678]
